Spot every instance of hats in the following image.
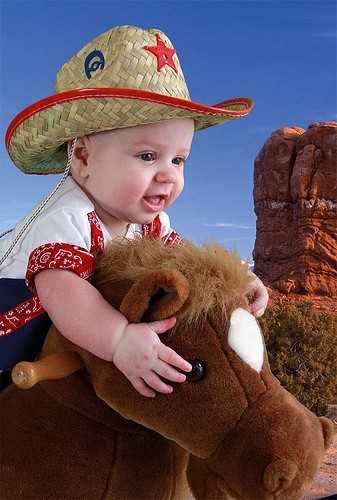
[3,24,253,175]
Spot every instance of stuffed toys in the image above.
[0,232,334,500]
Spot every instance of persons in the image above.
[0,24,268,398]
